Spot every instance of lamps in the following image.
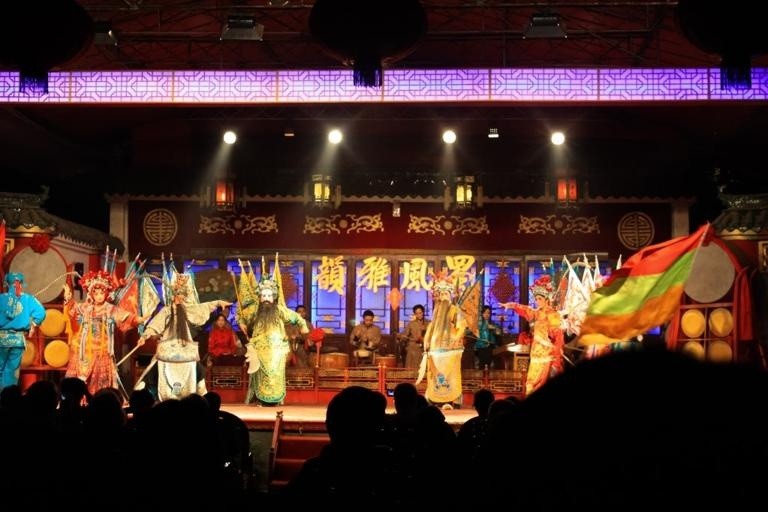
[215,180,236,212]
[311,174,332,205]
[556,172,580,210]
[451,176,476,210]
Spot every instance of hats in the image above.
[78,270,118,303]
[3,272,27,288]
[169,273,190,301]
[254,272,279,300]
[431,271,455,294]
[529,275,554,301]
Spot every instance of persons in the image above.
[0,242,768,512]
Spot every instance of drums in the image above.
[308,352,349,369]
[5,241,68,304]
[43,339,70,367]
[375,356,397,368]
[708,307,734,338]
[682,341,704,360]
[218,353,244,366]
[39,309,65,337]
[684,239,736,303]
[20,339,35,367]
[680,308,705,338]
[353,350,371,358]
[707,340,732,363]
[129,329,156,359]
